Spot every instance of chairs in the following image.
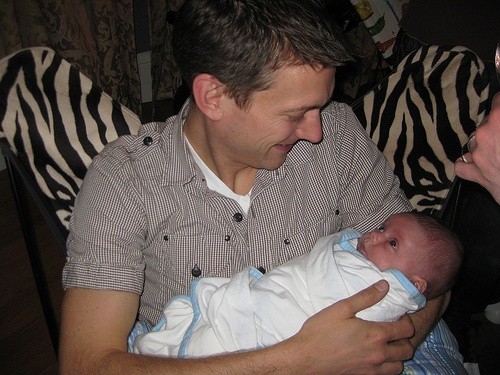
[0,44,490,362]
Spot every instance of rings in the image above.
[467,134,476,152]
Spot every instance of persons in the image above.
[57,1,467,375]
[454,90,500,205]
[131,211,463,358]
